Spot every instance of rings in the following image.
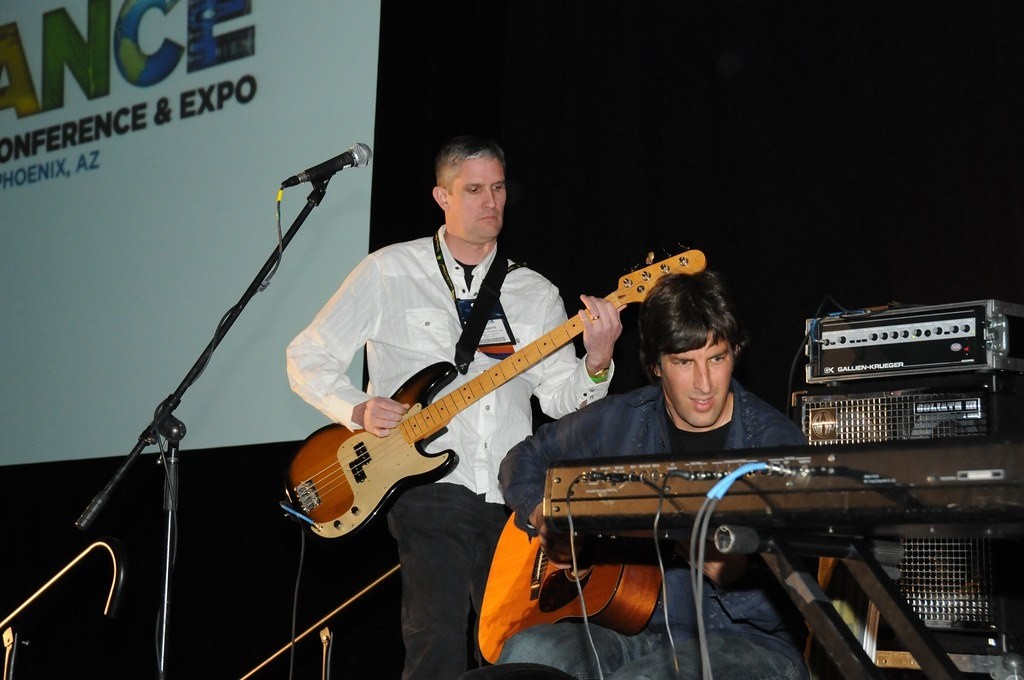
[591,316,599,320]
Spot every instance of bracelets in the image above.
[590,376,607,382]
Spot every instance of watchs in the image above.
[586,366,609,377]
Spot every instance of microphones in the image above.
[281,142,372,188]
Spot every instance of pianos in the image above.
[540,422,1024,570]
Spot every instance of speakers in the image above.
[787,371,1024,655]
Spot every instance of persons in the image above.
[495,272,808,680]
[285,140,627,680]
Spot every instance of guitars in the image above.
[476,507,668,669]
[282,237,709,542]
